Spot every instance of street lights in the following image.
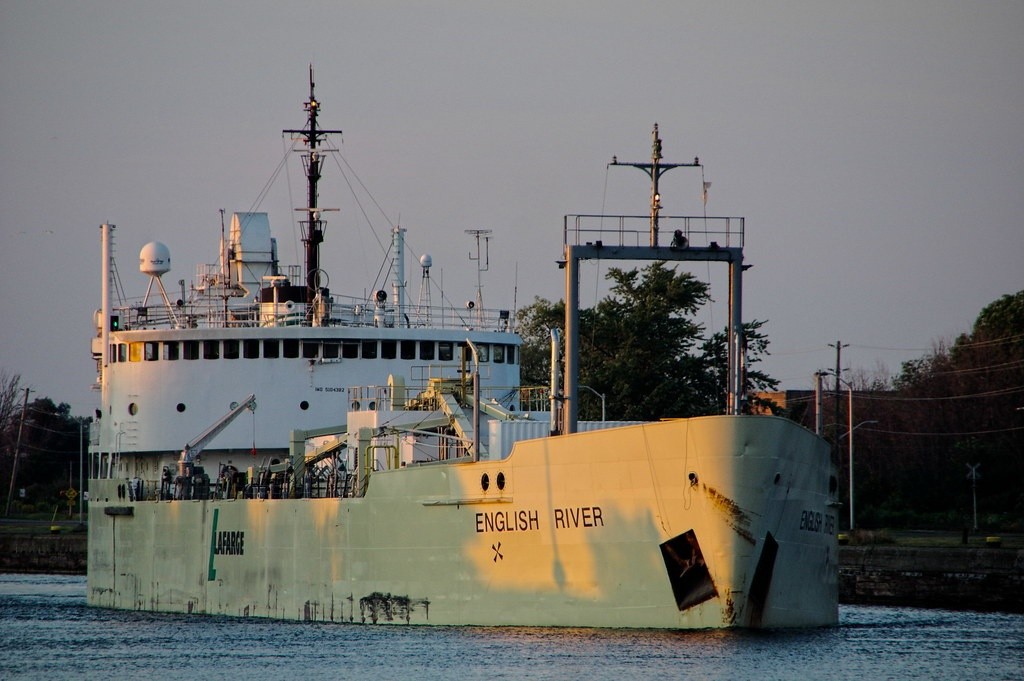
[821,372,855,532]
[54,412,83,523]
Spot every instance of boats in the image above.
[33,62,854,627]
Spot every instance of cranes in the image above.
[174,392,259,489]
